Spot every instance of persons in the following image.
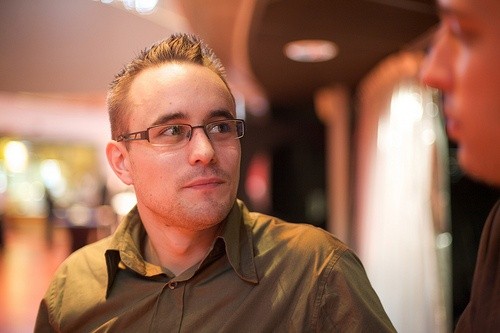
[417,0,499,333]
[31,31,396,333]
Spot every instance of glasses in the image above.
[114,118,246,151]
